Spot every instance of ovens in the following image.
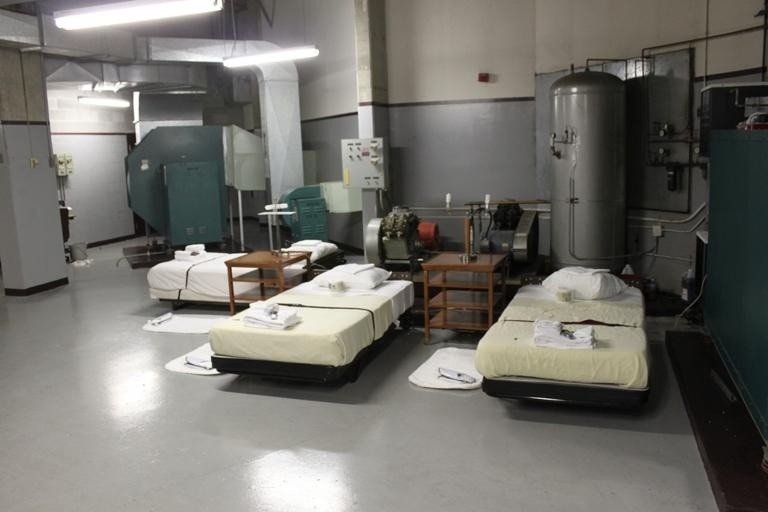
[146,247,346,312]
[477,283,651,418]
[207,275,415,388]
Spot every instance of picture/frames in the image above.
[224,248,312,317]
[421,250,508,342]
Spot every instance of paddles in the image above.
[409,345,483,390]
[141,312,231,375]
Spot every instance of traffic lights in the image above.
[310,262,392,291]
[283,239,337,264]
[541,266,628,300]
